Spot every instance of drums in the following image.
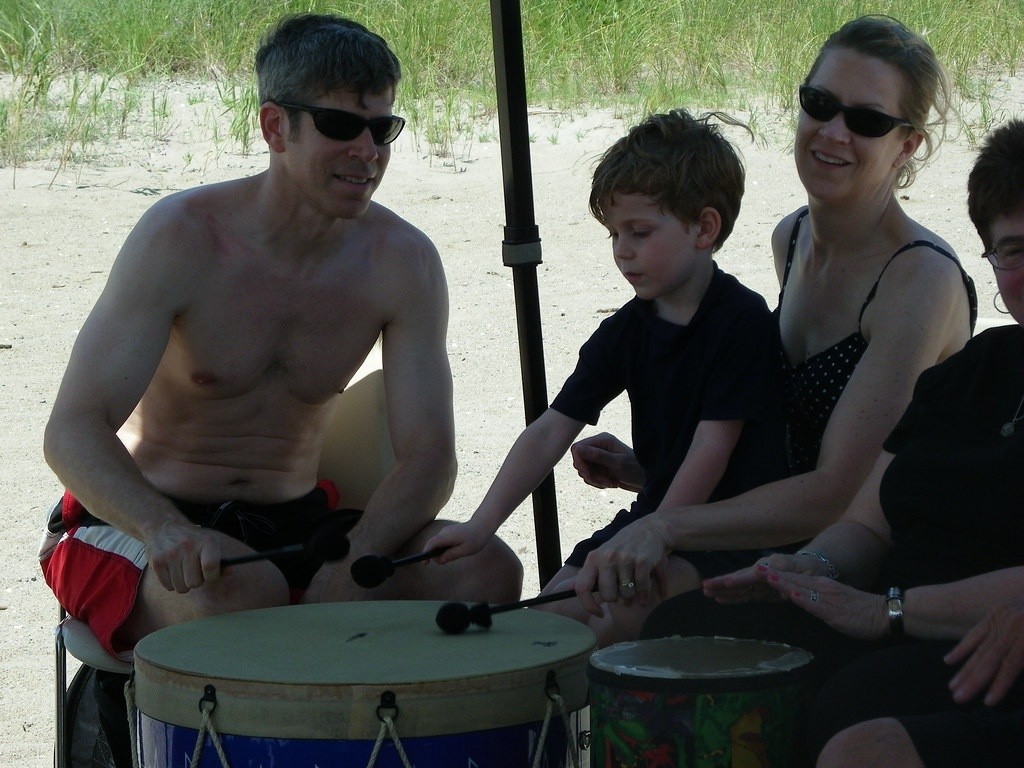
[134,600,599,768]
[571,631,815,768]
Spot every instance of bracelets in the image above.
[797,550,840,580]
[885,587,906,634]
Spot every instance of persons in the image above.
[418,109,791,656]
[703,121,1024,768]
[572,10,976,671]
[40,16,524,669]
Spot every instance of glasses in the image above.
[982,243,1024,270]
[277,103,405,145]
[799,85,914,137]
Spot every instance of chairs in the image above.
[53,370,396,768]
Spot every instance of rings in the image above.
[762,562,768,567]
[812,594,819,602]
[620,580,634,589]
